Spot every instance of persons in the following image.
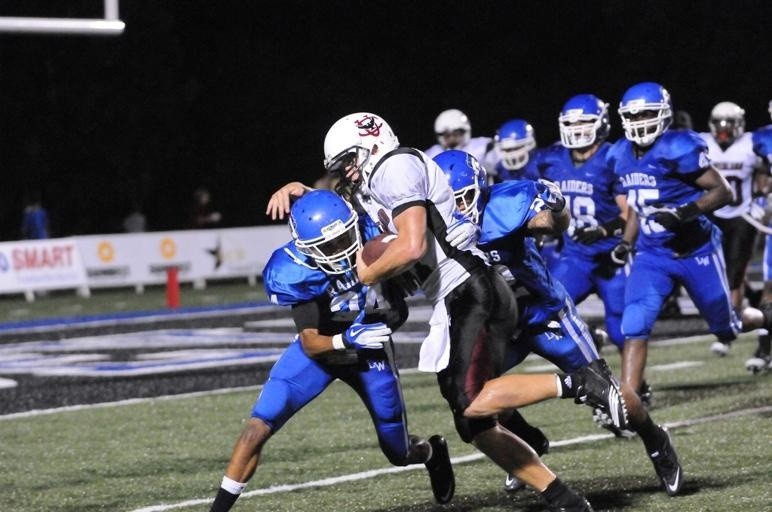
[518,94,651,402]
[421,108,500,177]
[203,191,455,512]
[265,112,627,512]
[747,96,772,373]
[592,83,772,438]
[429,148,684,497]
[494,121,606,357]
[695,100,772,357]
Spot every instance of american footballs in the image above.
[364,233,397,265]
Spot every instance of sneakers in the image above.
[647,426,684,496]
[554,495,595,512]
[427,434,455,505]
[745,357,767,378]
[504,426,550,491]
[591,406,640,442]
[569,365,630,431]
[710,340,732,357]
[589,327,608,352]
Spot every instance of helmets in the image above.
[493,118,538,171]
[557,93,612,152]
[431,150,490,220]
[708,101,746,144]
[321,111,401,201]
[288,187,366,275]
[617,82,673,148]
[752,123,768,166]
[434,108,472,153]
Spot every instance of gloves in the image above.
[656,210,684,232]
[572,221,606,248]
[445,211,483,250]
[340,309,392,351]
[401,271,421,299]
[609,239,632,269]
